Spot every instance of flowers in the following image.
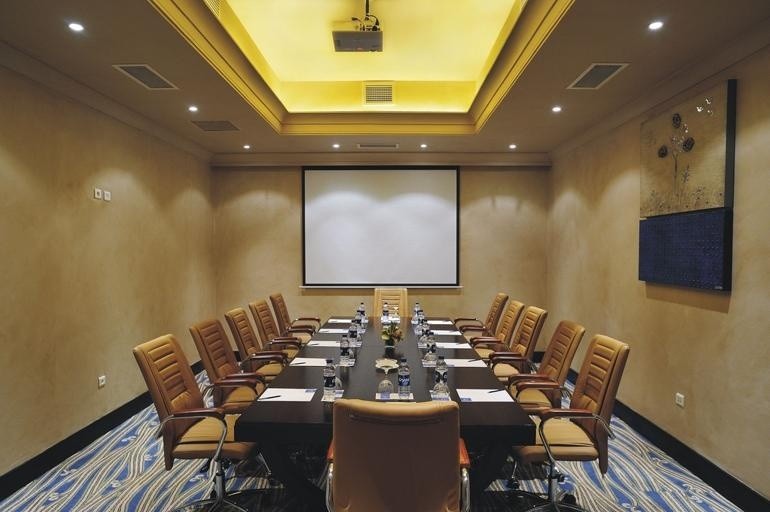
[380,321,403,342]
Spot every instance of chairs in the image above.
[249,298,313,359]
[189,318,285,483]
[225,305,301,383]
[326,398,471,512]
[374,288,408,316]
[133,334,259,512]
[472,305,548,385]
[492,319,586,415]
[511,333,631,511]
[454,292,508,342]
[461,300,524,363]
[270,293,321,344]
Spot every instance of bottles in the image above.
[323,302,365,402]
[383,303,388,322]
[398,358,410,398]
[414,302,448,399]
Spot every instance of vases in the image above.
[384,338,396,348]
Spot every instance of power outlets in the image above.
[676,392,684,406]
[99,375,106,388]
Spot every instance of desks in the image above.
[234,317,536,511]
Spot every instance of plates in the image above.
[375,357,400,370]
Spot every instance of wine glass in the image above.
[377,365,394,400]
[392,306,400,324]
[334,312,369,395]
[412,311,450,395]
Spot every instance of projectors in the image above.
[331,21,383,52]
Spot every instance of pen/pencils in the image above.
[468,358,481,362]
[259,395,280,400]
[322,331,328,332]
[311,344,318,345]
[488,388,504,393]
[290,362,305,365]
